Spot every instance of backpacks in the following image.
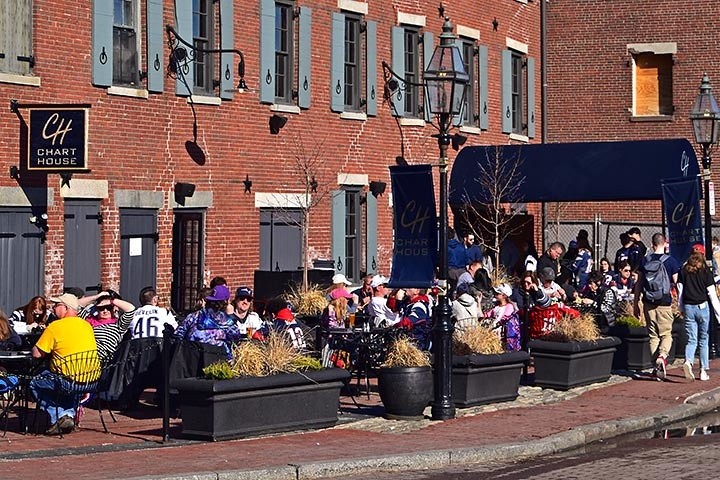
[643,253,670,302]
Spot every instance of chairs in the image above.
[0,302,581,438]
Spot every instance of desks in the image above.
[0,351,33,438]
[329,329,386,400]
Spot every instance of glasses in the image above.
[624,269,631,272]
[97,304,113,312]
[240,295,252,302]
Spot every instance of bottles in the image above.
[349,312,355,327]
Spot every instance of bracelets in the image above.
[634,299,640,302]
[110,298,115,306]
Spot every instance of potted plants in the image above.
[526,308,621,391]
[451,317,529,408]
[378,333,435,420]
[174,317,353,441]
[614,315,680,370]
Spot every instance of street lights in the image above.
[421,15,470,421]
[688,70,720,359]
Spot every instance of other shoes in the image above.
[683,360,695,382]
[699,370,709,380]
[45,417,75,436]
[653,356,667,382]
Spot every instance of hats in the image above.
[492,283,512,297]
[540,267,555,280]
[691,244,705,255]
[235,286,253,297]
[332,274,352,285]
[524,255,537,272]
[329,288,353,300]
[626,227,641,234]
[276,308,293,320]
[50,293,79,311]
[205,286,230,301]
[369,274,390,287]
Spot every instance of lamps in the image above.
[165,24,261,94]
[29,214,49,232]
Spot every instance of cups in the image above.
[246,327,254,339]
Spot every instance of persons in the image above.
[0,228,720,436]
